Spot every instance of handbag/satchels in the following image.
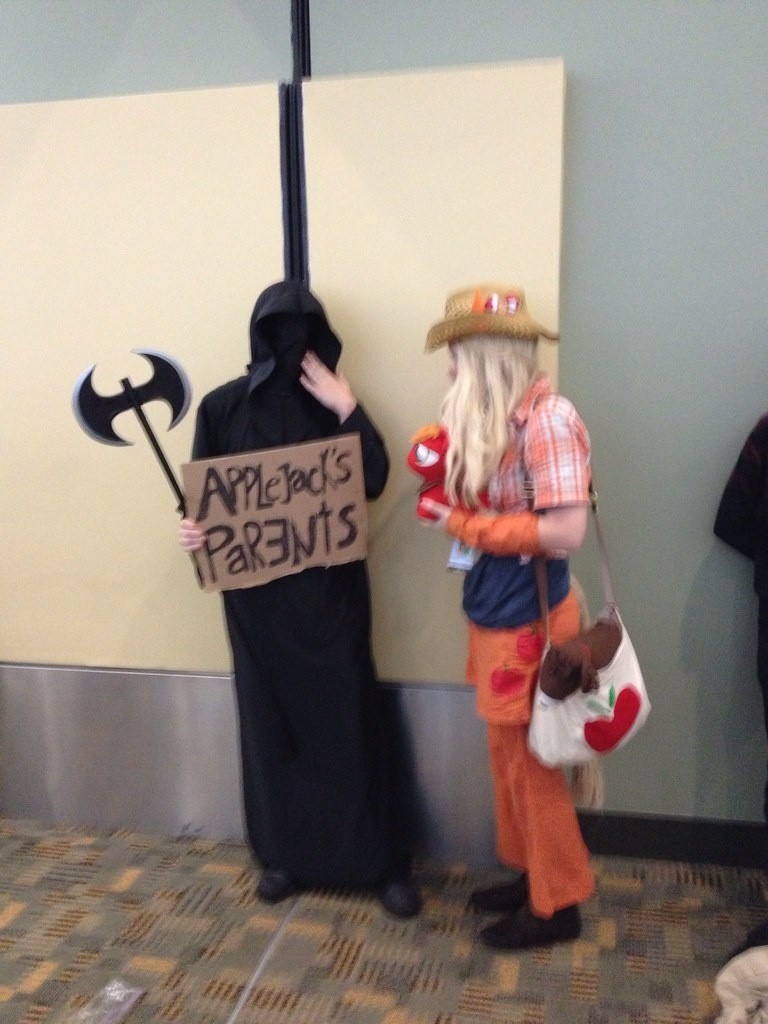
[526,606,652,768]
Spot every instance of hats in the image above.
[425,286,561,353]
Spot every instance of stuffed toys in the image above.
[406,426,490,521]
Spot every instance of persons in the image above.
[417,287,598,949]
[178,281,429,921]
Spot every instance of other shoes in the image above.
[258,869,305,899]
[372,876,418,916]
[472,873,530,912]
[480,902,583,949]
